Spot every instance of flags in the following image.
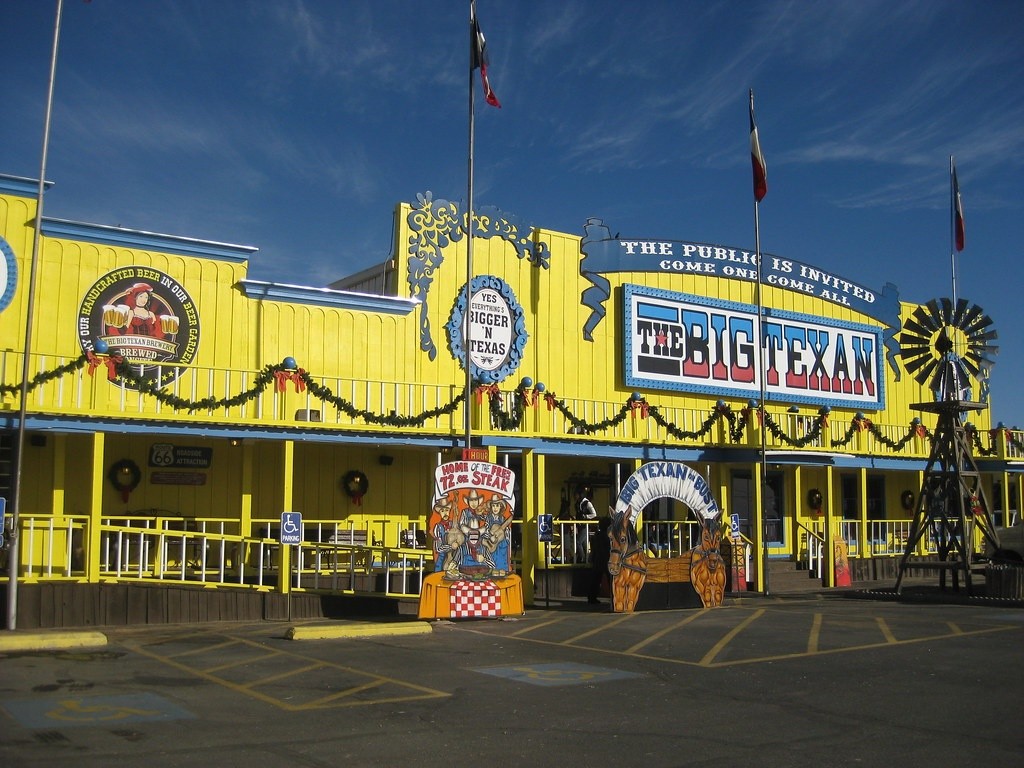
[749,95,768,201]
[471,14,502,108]
[952,166,965,251]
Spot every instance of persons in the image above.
[574,484,597,564]
